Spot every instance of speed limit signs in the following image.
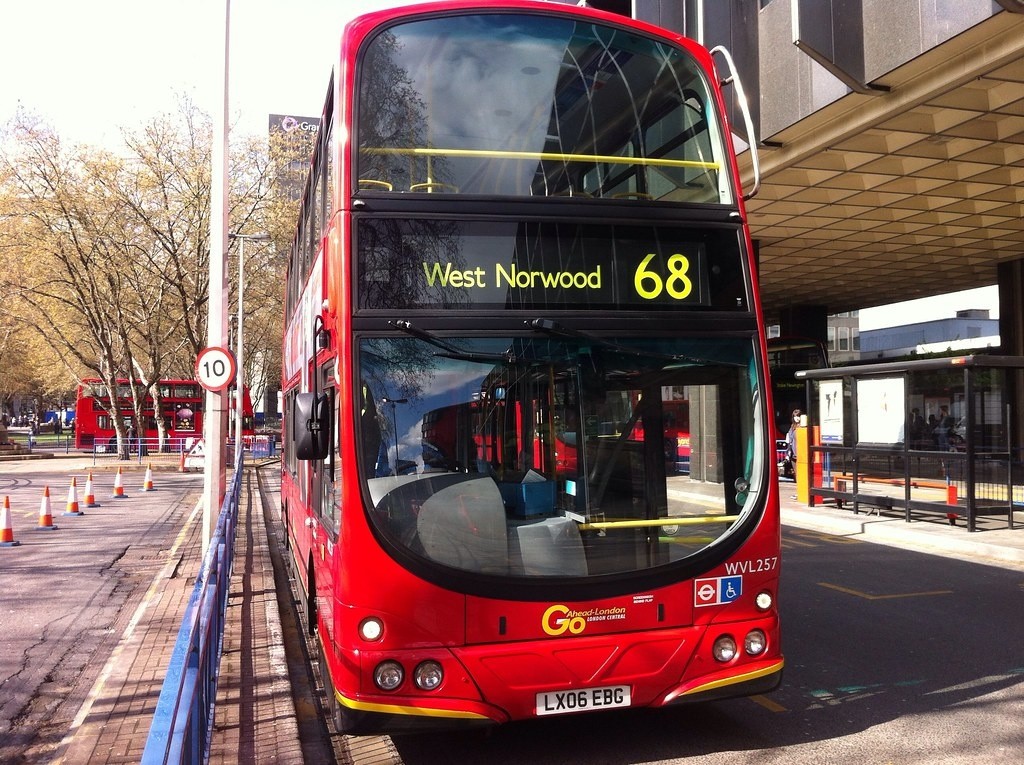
[195,346,236,393]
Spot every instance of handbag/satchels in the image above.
[783,460,795,479]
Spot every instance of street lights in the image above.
[229,230,275,473]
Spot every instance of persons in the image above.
[908,405,939,460]
[786,410,802,477]
[28,421,37,446]
[71,418,75,438]
[0,414,11,446]
[933,405,958,477]
[125,424,136,453]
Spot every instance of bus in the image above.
[74,378,256,454]
[283,0,790,735]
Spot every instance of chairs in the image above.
[358,179,656,201]
[374,435,557,518]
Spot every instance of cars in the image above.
[628,398,688,473]
[601,420,623,435]
[951,417,966,446]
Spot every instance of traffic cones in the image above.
[178,448,191,473]
[138,462,157,491]
[34,485,58,530]
[110,466,128,498]
[0,495,21,546]
[81,470,101,508]
[60,477,84,517]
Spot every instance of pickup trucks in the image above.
[32,411,60,435]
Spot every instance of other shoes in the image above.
[944,476,952,480]
